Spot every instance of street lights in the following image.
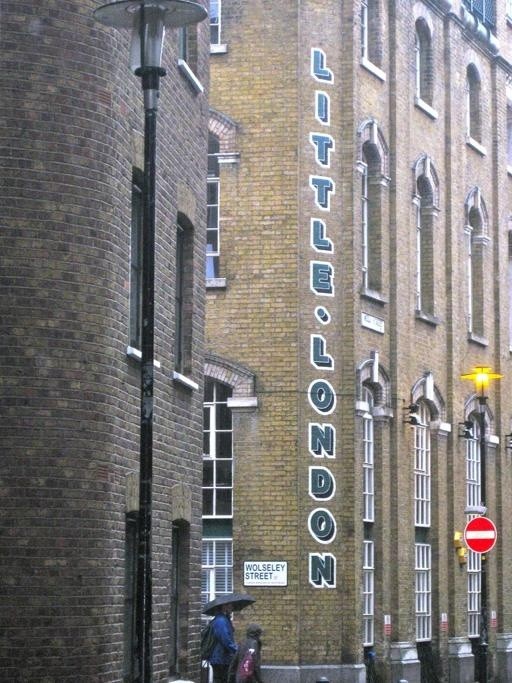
[91,0,211,680]
[461,365,504,683]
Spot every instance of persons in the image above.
[224,622,264,683]
[207,601,240,682]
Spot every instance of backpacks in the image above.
[200,619,217,659]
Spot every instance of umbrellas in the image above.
[201,592,257,622]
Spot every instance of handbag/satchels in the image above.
[240,648,255,680]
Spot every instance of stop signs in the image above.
[463,517,498,553]
